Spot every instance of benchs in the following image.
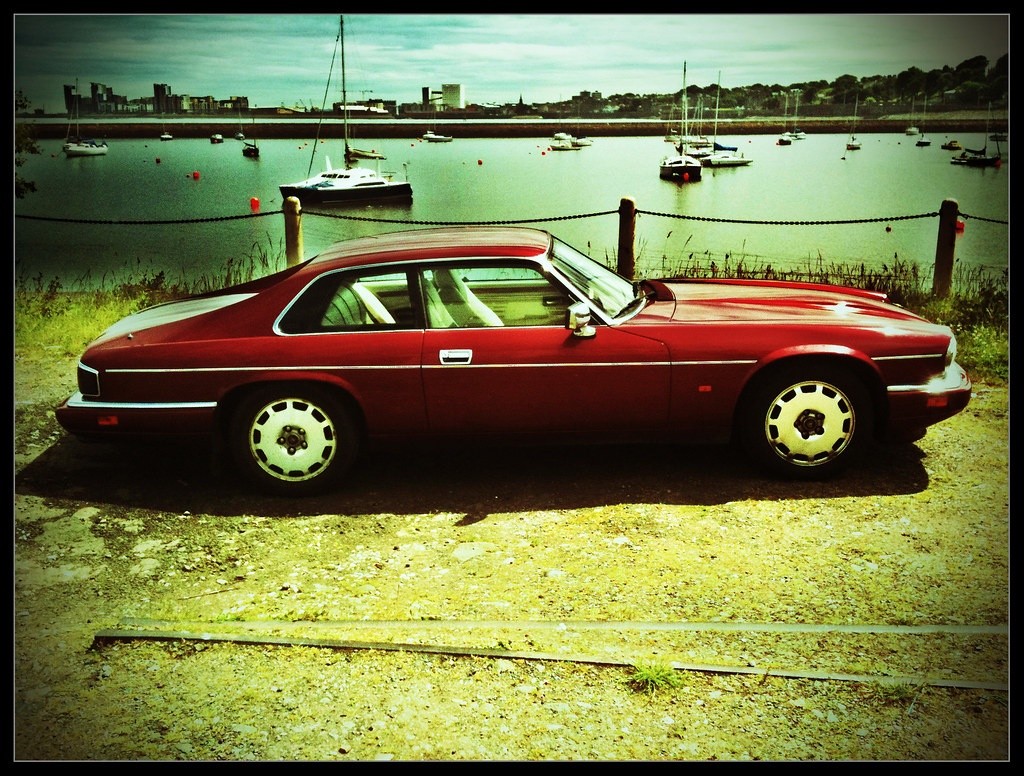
[323,283,397,326]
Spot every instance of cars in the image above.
[53,225,973,496]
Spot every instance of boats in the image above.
[210,134,223,144]
[941,140,964,151]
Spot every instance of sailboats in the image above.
[549,94,594,150]
[988,95,1008,141]
[234,107,245,140]
[950,99,1003,170]
[846,93,862,150]
[777,94,792,146]
[277,14,414,208]
[422,103,453,143]
[62,77,110,157]
[160,111,174,141]
[904,94,919,135]
[241,110,260,158]
[347,107,388,159]
[663,70,754,167]
[781,89,807,140]
[659,58,702,185]
[915,94,932,147]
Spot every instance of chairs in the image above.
[423,279,466,329]
[432,270,503,334]
